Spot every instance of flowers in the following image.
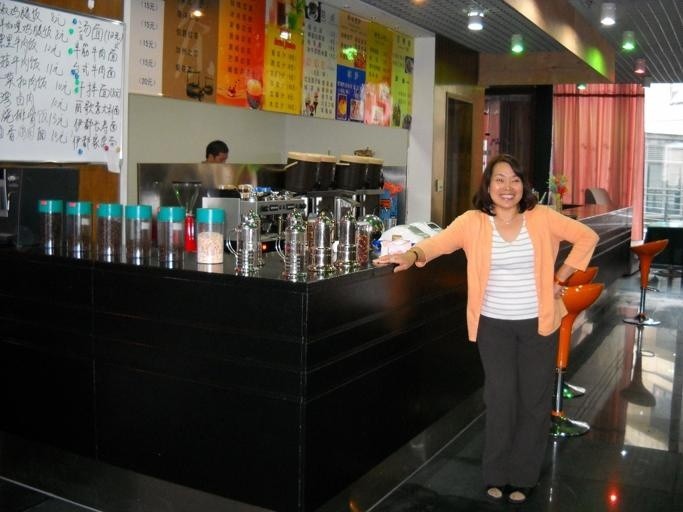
[544,174,572,200]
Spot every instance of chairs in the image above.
[585,184,612,204]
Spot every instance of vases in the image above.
[551,195,562,210]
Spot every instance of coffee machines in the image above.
[203,185,310,254]
[172,179,202,216]
[1,165,80,253]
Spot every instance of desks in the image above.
[644,218,681,291]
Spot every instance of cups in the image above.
[247,80,263,111]
[337,94,364,122]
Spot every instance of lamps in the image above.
[577,75,589,91]
[632,58,646,78]
[467,6,484,29]
[509,31,525,53]
[597,2,616,26]
[621,31,634,52]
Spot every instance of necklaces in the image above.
[496,212,519,225]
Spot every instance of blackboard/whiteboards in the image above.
[0,0,126,165]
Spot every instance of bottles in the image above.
[225,210,375,278]
[36,198,224,264]
[393,103,401,126]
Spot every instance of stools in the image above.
[622,236,668,327]
[617,325,657,409]
[551,282,605,439]
[550,266,601,403]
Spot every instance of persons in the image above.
[370,149,604,506]
[193,140,232,166]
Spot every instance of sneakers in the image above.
[507,482,536,504]
[484,483,504,502]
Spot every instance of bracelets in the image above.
[554,278,566,289]
[407,246,418,264]
